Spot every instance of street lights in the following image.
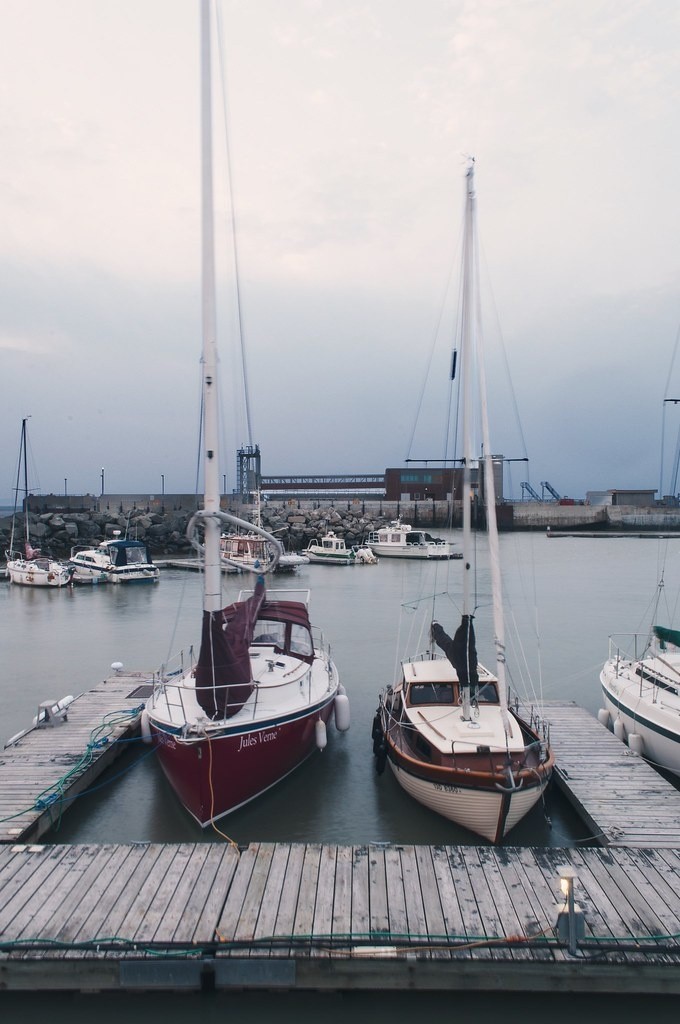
[374,157,561,844]
[222,474,227,494]
[161,474,165,494]
[100,474,104,494]
[64,479,67,496]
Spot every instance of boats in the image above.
[300,530,356,564]
[360,513,464,559]
[68,540,160,584]
[166,481,310,573]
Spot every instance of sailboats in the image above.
[140,0,350,829]
[5,417,71,587]
[595,330,680,785]
